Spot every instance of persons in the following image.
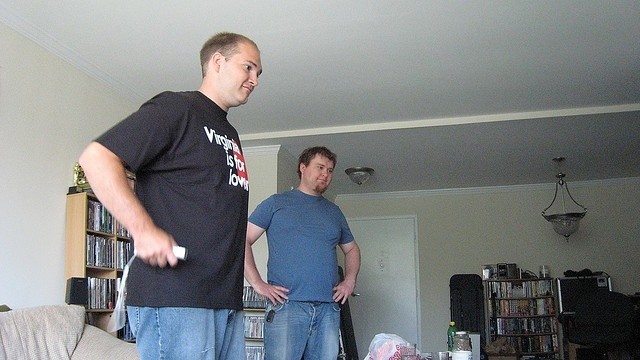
[80,32,263,359]
[244,147,360,360]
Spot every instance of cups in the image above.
[538,264,551,279]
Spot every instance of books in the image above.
[243,287,264,359]
[88,199,134,312]
[487,280,559,359]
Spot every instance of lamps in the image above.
[345,167,374,185]
[540,156,587,243]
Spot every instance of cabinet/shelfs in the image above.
[239,282,273,360]
[66,193,137,344]
[482,262,562,360]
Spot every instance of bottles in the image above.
[451,330,474,360]
[447,320,459,360]
